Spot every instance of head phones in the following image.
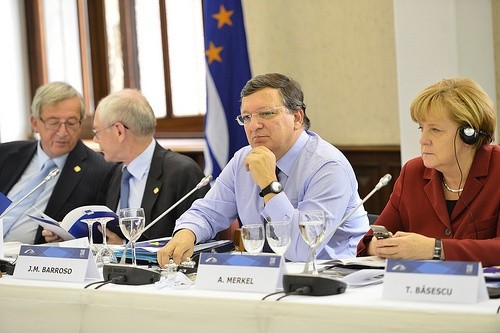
[460,125,493,144]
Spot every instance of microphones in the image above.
[282,174,394,295]
[0,170,59,218]
[103,176,213,284]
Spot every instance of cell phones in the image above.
[370,224,392,241]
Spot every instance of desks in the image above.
[0,258,500,333]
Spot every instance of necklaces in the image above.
[443,174,463,192]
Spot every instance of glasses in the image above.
[235,106,289,126]
[93,122,128,139]
[40,116,81,129]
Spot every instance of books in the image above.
[26,205,119,241]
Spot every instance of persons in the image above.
[42,88,211,266]
[157,73,370,269]
[356,77,500,268]
[0,81,120,245]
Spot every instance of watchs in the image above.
[432,238,442,260]
[259,180,283,197]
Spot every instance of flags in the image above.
[202,0,252,187]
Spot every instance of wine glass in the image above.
[242,224,265,254]
[80,219,99,261]
[120,207,146,267]
[298,210,325,273]
[265,221,292,255]
[95,216,118,278]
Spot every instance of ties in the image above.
[119,166,132,217]
[263,167,280,252]
[2,158,56,238]
